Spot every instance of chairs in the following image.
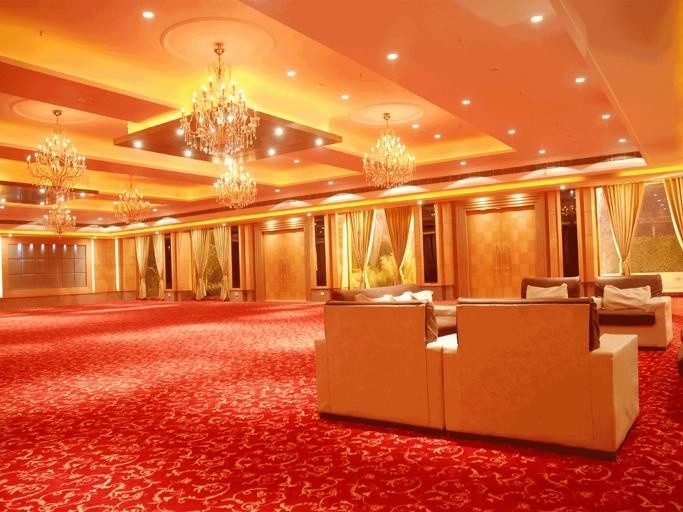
[517,274,673,350]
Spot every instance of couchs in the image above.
[444,299,639,457]
[318,299,458,435]
[327,283,459,338]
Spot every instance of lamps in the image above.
[363,111,417,190]
[114,172,151,223]
[25,107,90,235]
[176,39,264,207]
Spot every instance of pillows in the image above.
[360,289,434,304]
